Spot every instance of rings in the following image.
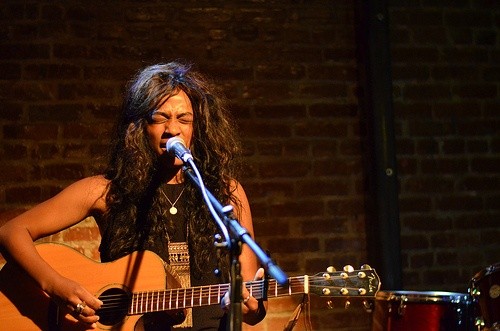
[76,301,86,314]
[243,294,250,303]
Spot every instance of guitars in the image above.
[0,243,382,331]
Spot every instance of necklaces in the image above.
[161,186,185,214]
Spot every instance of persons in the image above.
[0,62,269,331]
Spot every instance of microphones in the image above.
[166,136,193,162]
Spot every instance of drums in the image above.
[469,262,500,331]
[372,290,479,331]
